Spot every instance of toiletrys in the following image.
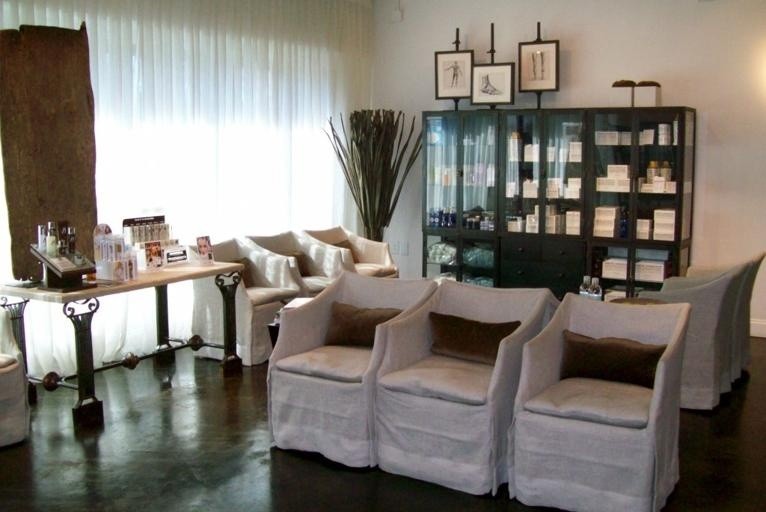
[428,124,495,232]
[36,219,78,256]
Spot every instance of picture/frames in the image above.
[470,61,515,106]
[434,49,474,100]
[518,38,560,94]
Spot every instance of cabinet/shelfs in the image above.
[587,105,697,298]
[500,107,588,301]
[421,109,500,289]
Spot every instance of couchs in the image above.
[0,307,32,451]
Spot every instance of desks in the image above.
[1,261,247,436]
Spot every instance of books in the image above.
[505,120,694,302]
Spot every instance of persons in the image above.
[149,242,159,263]
[197,238,209,255]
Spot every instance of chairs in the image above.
[300,224,400,281]
[375,277,563,496]
[661,249,766,382]
[186,235,302,368]
[608,263,751,411]
[267,268,437,469]
[240,230,343,302]
[506,292,692,512]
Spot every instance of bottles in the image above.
[37,220,75,256]
[461,212,480,230]
[660,161,672,183]
[580,274,602,300]
[510,132,523,163]
[647,160,660,185]
[426,206,456,227]
